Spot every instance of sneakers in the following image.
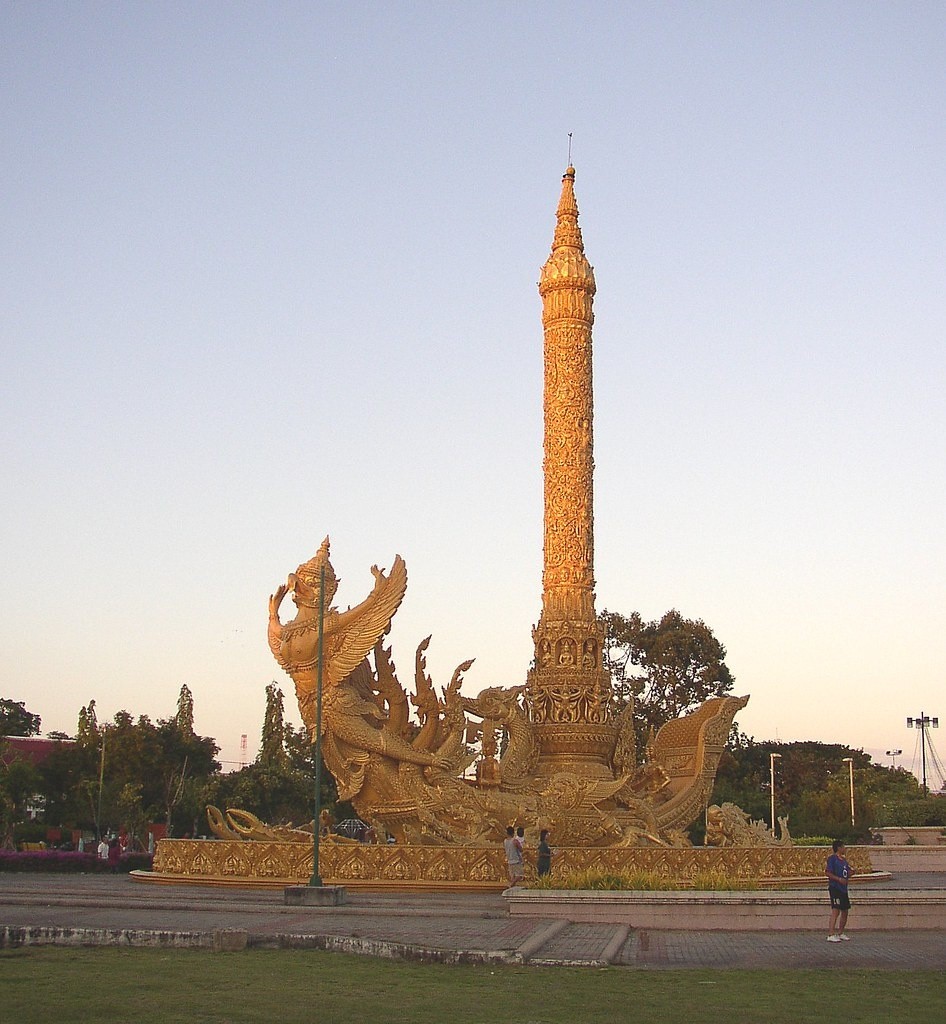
[827,934,842,943]
[838,933,850,941]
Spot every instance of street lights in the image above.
[843,756,855,827]
[769,753,782,838]
[885,750,902,772]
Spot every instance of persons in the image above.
[637,725,671,807]
[538,829,554,880]
[522,640,608,724]
[825,841,855,943]
[503,826,524,888]
[98,836,121,876]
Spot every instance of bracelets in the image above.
[838,877,839,882]
